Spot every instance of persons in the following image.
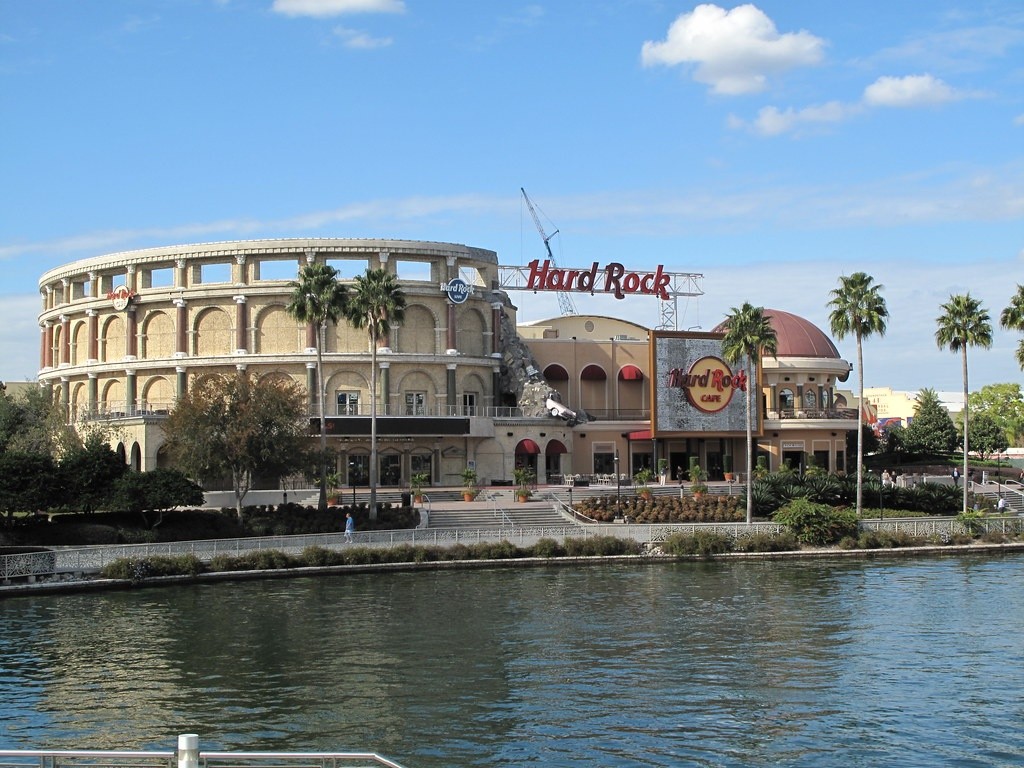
[882,470,889,486]
[952,467,960,487]
[998,497,1005,513]
[677,466,684,485]
[890,471,897,488]
[1020,471,1024,481]
[660,464,667,486]
[344,513,354,543]
[972,502,980,510]
[968,468,973,488]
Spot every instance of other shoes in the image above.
[350,541,353,544]
[345,541,349,543]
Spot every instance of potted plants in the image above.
[326,472,343,505]
[657,459,668,483]
[411,473,428,503]
[723,455,733,480]
[687,465,709,498]
[511,468,537,502]
[635,487,653,499]
[458,469,478,502]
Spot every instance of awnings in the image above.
[543,364,568,380]
[516,440,541,454]
[618,365,643,380]
[581,365,606,379]
[546,439,567,453]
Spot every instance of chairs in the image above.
[564,473,625,485]
